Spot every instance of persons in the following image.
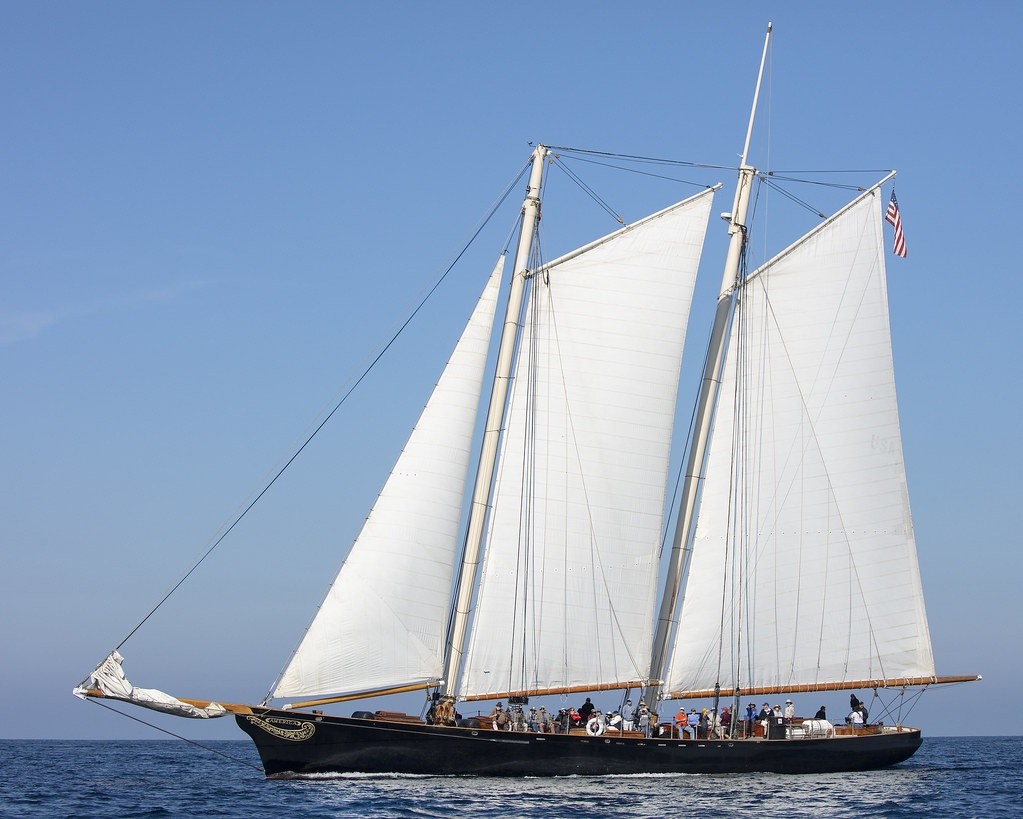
[860,702,868,724]
[850,693,859,710]
[815,706,825,720]
[491,695,793,739]
[849,705,863,728]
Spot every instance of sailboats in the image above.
[83,19,989,775]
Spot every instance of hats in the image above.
[496,694,865,712]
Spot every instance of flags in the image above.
[885,189,907,257]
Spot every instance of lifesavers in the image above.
[586,718,604,736]
